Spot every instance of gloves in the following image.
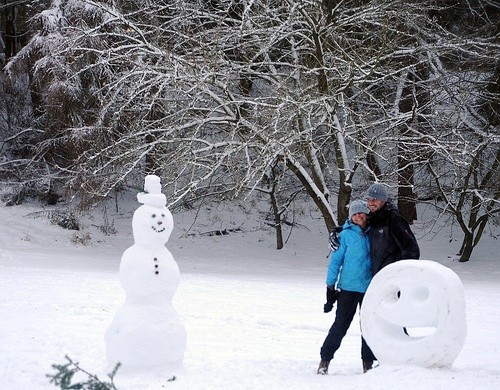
[328,229,340,252]
[324,288,334,302]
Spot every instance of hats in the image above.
[366,184,388,201]
[349,200,370,220]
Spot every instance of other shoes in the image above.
[363,361,372,373]
[317,358,330,375]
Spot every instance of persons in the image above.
[317,183,420,375]
